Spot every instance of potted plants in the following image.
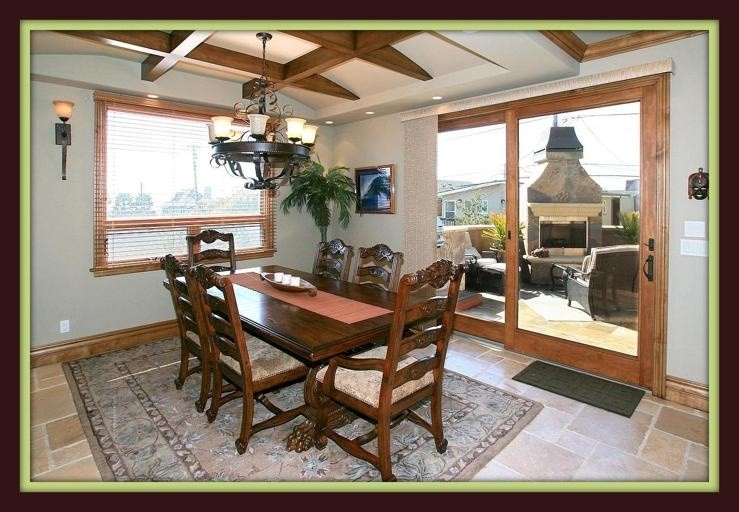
[481,212,528,263]
[355,164,396,214]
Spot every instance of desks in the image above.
[552,263,584,296]
[163,265,430,452]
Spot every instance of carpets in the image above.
[510,359,646,418]
[62,322,545,482]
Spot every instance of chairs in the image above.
[565,243,641,321]
[184,264,310,455]
[444,229,499,287]
[314,257,465,479]
[355,244,403,294]
[312,239,355,282]
[186,230,235,276]
[162,254,237,414]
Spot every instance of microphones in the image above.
[481,262,522,294]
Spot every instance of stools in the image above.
[481,262,522,294]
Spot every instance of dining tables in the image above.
[481,212,528,263]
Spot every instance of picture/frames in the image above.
[552,263,584,296]
[355,164,396,214]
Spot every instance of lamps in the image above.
[444,229,499,287]
[565,243,641,321]
[201,31,320,192]
[50,99,75,180]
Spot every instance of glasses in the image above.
[50,99,75,180]
[201,31,320,192]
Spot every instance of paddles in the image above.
[62,322,545,482]
[510,359,646,418]
